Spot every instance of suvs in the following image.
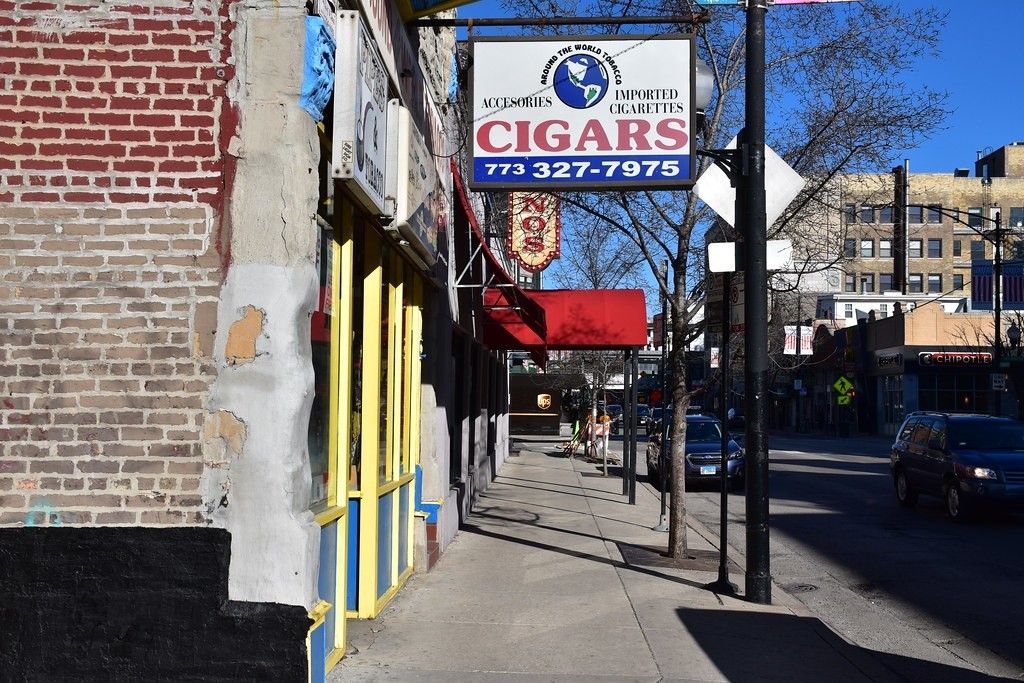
[646,409,745,487]
[889,409,1024,515]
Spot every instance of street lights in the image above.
[868,196,1021,417]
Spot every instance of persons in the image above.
[727,406,736,422]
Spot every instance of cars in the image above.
[646,407,672,436]
[630,403,649,427]
[606,404,624,423]
